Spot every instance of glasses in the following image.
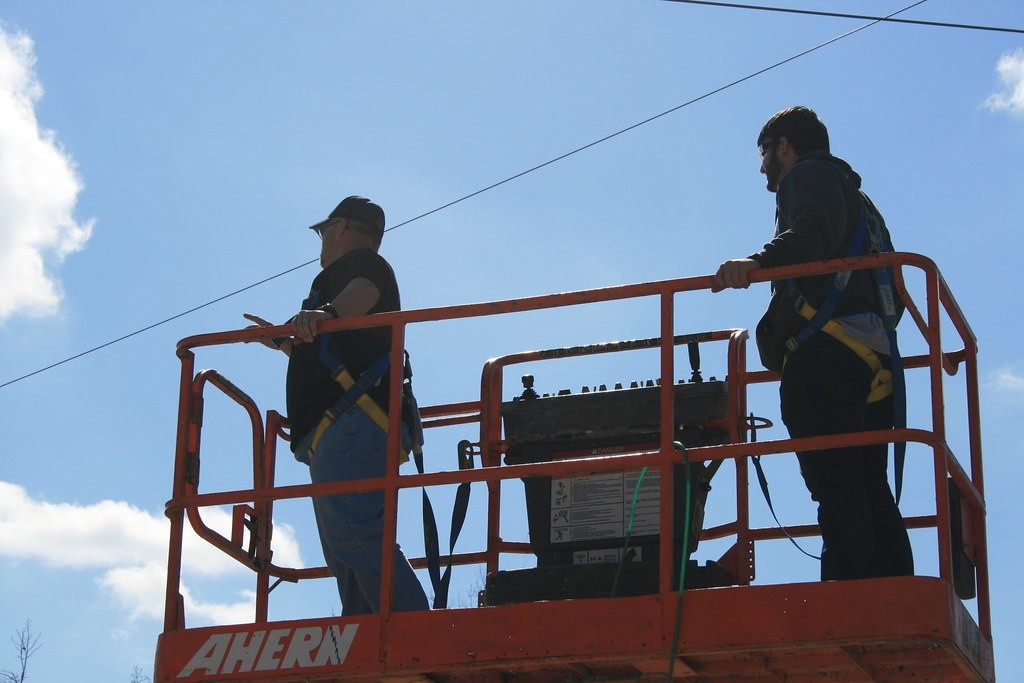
[315,216,349,240]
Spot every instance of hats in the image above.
[309,195,385,234]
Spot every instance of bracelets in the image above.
[318,302,338,319]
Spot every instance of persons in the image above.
[712,106,916,581]
[244,195,430,618]
